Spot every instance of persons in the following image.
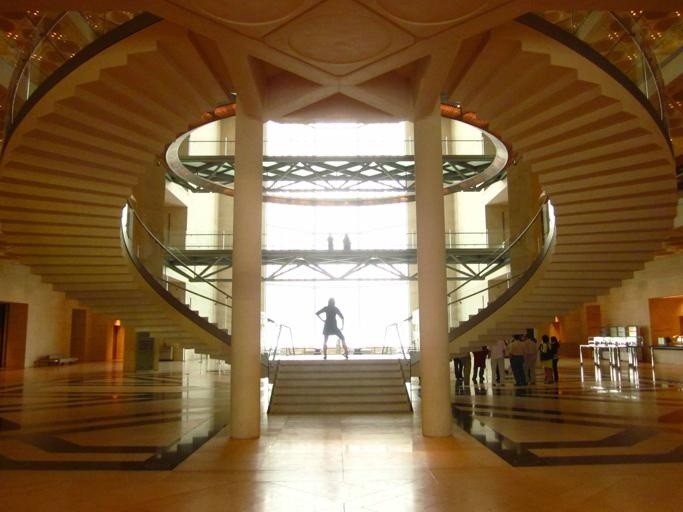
[448,332,560,386]
[326,234,333,250]
[343,233,350,250]
[316,297,348,358]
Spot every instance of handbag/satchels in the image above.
[539,342,553,360]
